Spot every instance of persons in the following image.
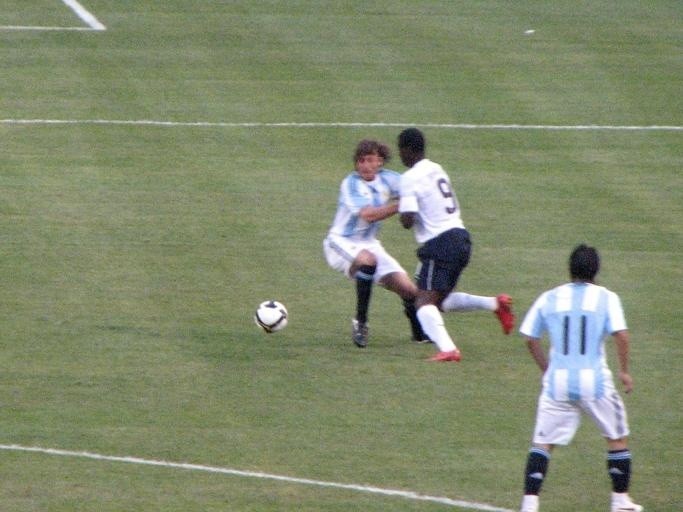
[319,138,434,348]
[511,242,648,512]
[394,127,515,365]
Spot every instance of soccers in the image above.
[255,301,289,334]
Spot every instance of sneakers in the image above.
[610,491,644,512]
[521,494,539,512]
[410,336,431,343]
[352,319,369,347]
[494,294,516,335]
[423,349,461,362]
